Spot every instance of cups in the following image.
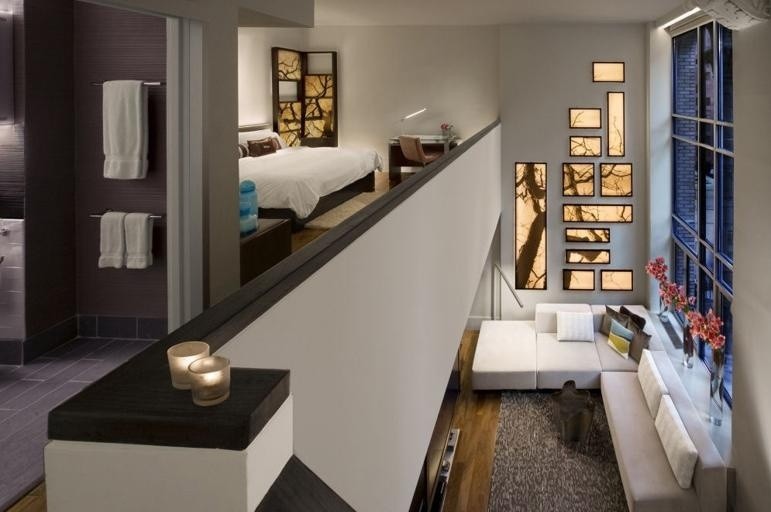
[190,355,231,407]
[166,340,209,392]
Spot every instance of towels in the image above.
[102,79,148,179]
[125,214,155,269]
[98,212,126,270]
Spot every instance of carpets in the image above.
[489,389,629,511]
[291,226,329,253]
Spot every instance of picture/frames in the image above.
[592,62,625,82]
[515,162,547,290]
[561,91,633,291]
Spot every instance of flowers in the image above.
[660,282,696,313]
[646,256,670,283]
[685,309,726,349]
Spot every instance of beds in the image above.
[238,124,383,230]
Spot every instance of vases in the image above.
[710,363,722,426]
[660,283,668,323]
[683,326,692,368]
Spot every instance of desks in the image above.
[239,218,293,287]
[388,135,456,189]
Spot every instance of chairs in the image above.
[398,136,442,166]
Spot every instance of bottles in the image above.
[240,179,258,222]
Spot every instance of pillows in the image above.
[238,129,287,158]
[654,394,699,490]
[556,312,594,341]
[600,305,651,362]
[637,349,668,418]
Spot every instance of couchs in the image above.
[473,304,727,512]
